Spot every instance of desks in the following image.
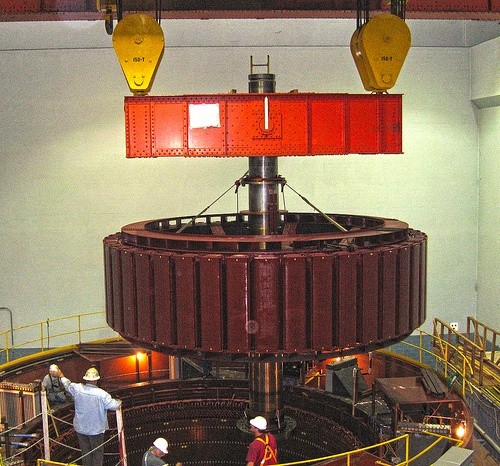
[372,375,461,451]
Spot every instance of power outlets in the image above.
[450,323,458,331]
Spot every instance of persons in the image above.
[245,416,278,466]
[53,368,120,466]
[42,363,69,406]
[142,437,182,466]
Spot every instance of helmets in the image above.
[250,416,267,430]
[83,368,100,380]
[49,364,58,371]
[153,438,168,454]
